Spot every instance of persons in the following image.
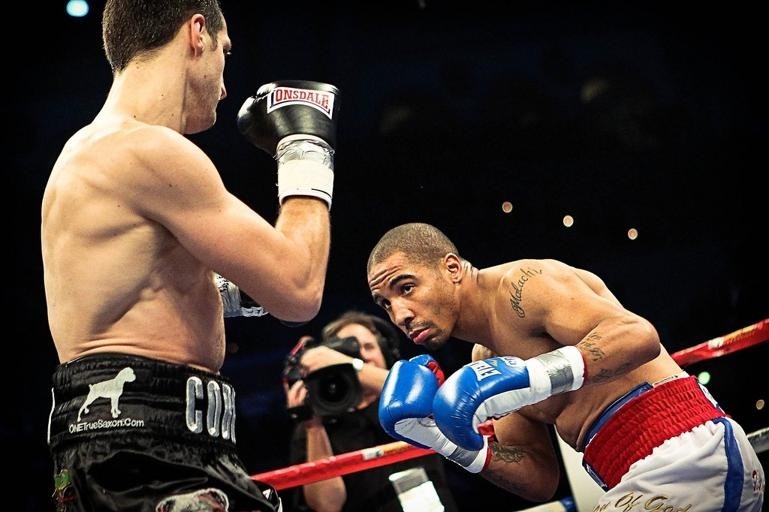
[287,310,457,511]
[366,220,768,510]
[39,0,342,509]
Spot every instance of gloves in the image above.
[378,355,488,474]
[214,273,269,317]
[235,80,341,210]
[433,346,584,451]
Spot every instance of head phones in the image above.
[373,316,403,370]
